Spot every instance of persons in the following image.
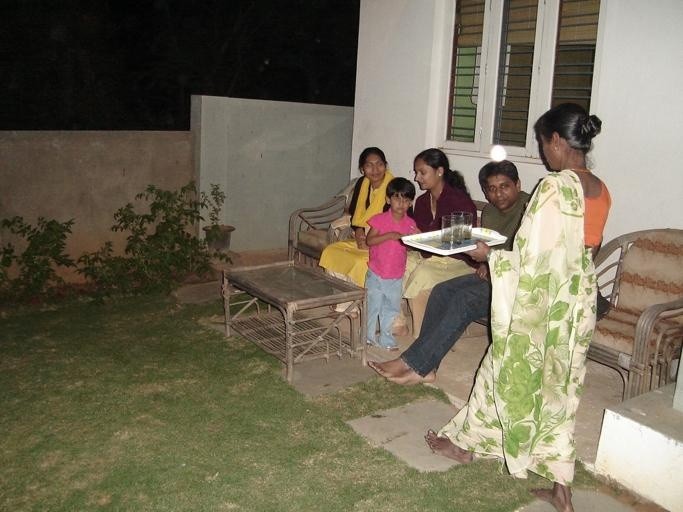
[363,177,422,352]
[365,159,528,386]
[390,147,479,340]
[316,145,414,317]
[424,102,612,512]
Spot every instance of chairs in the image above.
[287,177,358,269]
[587,227,683,402]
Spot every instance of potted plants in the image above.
[202,182,235,251]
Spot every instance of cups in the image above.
[440,211,473,245]
